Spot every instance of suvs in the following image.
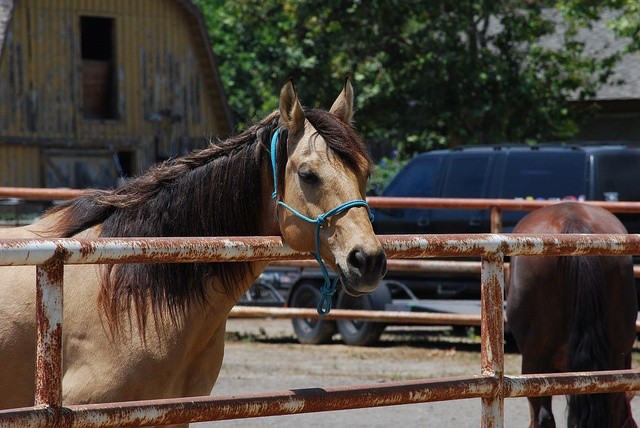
[284,145,640,345]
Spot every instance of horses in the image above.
[506,202,639,428]
[0,76,387,414]
